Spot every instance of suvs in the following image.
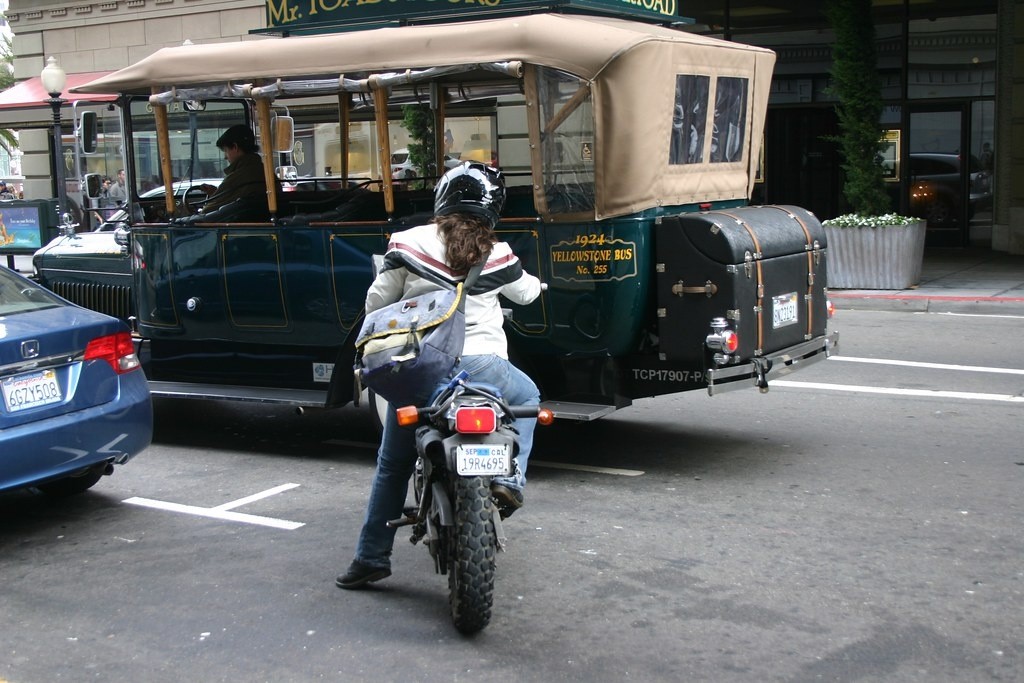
[882,151,995,247]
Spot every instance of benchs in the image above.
[169,171,596,227]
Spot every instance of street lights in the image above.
[42,56,75,240]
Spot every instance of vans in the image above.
[33,1,841,432]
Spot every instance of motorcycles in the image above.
[383,376,523,639]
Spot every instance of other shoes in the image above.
[488,482,524,507]
[335,558,392,586]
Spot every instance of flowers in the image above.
[823,209,921,228]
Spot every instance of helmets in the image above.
[433,160,506,229]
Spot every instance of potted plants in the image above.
[817,0,928,290]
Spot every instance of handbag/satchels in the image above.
[353,282,466,402]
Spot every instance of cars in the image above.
[94,178,297,239]
[373,146,464,186]
[0,264,153,509]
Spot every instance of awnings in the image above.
[0,69,120,110]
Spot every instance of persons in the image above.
[294,142,302,165]
[336,165,541,591]
[0,169,160,229]
[193,124,283,215]
[980,142,993,171]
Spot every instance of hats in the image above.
[0,181,6,186]
[215,124,255,149]
